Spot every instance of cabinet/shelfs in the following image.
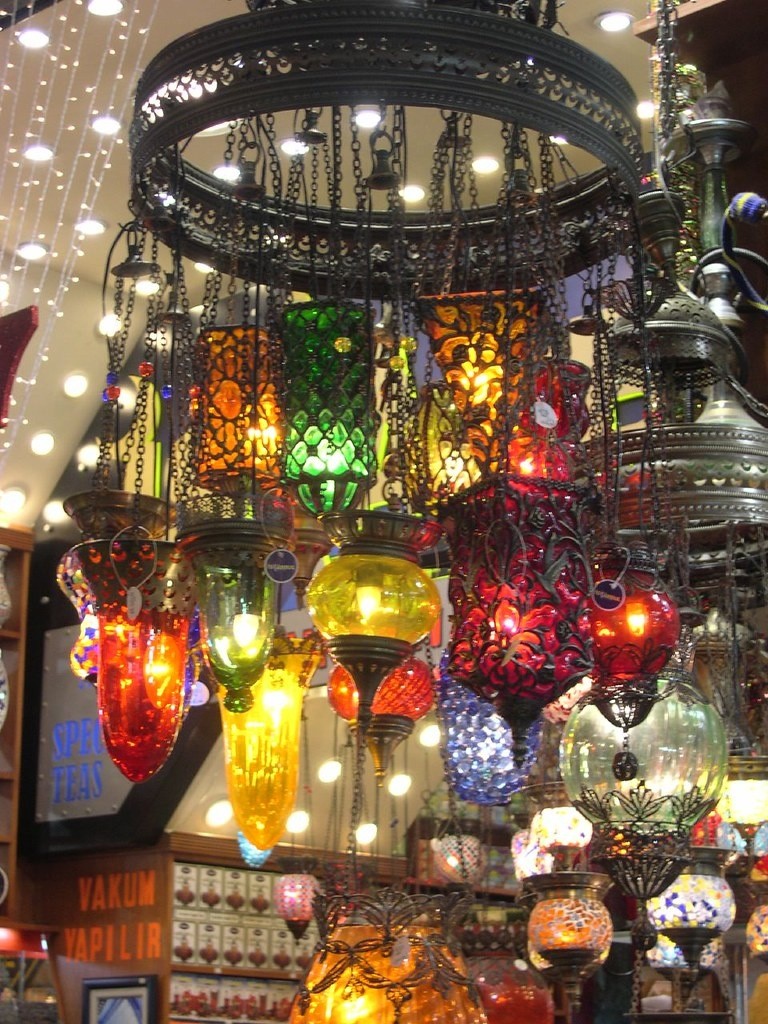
[586,844,748,1024]
[0,527,37,920]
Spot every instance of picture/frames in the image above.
[81,974,160,1024]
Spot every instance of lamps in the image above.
[58,0,768,1024]
